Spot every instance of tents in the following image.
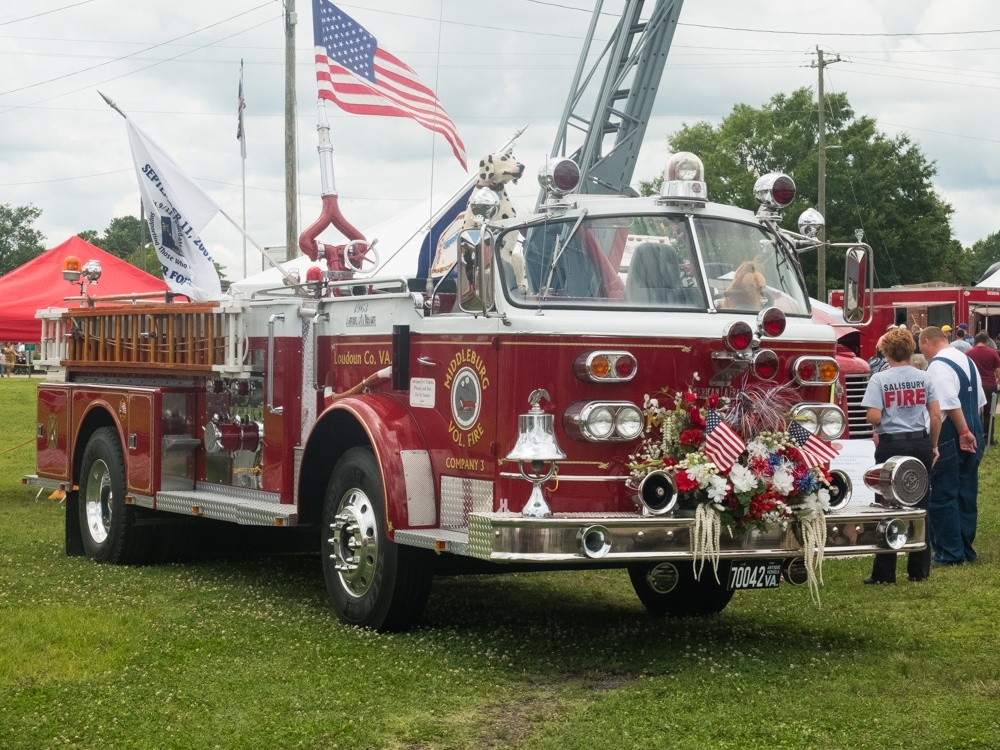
[0,235,191,343]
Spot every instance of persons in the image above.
[918,325,987,568]
[956,323,973,345]
[965,331,1000,445]
[861,327,942,585]
[941,325,953,336]
[911,324,922,354]
[876,324,899,348]
[0,342,25,377]
[950,330,972,353]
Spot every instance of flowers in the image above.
[625,371,833,610]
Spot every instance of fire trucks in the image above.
[828,262,1000,401]
[21,0,932,635]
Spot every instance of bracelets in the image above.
[959,429,969,435]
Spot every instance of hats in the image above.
[941,325,952,331]
[899,324,906,329]
[888,324,899,329]
[956,323,968,330]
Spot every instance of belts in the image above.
[879,431,924,441]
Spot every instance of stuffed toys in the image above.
[725,260,766,309]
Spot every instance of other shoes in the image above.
[908,574,926,581]
[931,560,963,568]
[863,577,896,583]
[990,440,998,445]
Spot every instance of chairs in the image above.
[624,243,684,302]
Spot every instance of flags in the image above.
[125,117,222,301]
[311,0,468,172]
[705,408,745,472]
[787,419,840,470]
